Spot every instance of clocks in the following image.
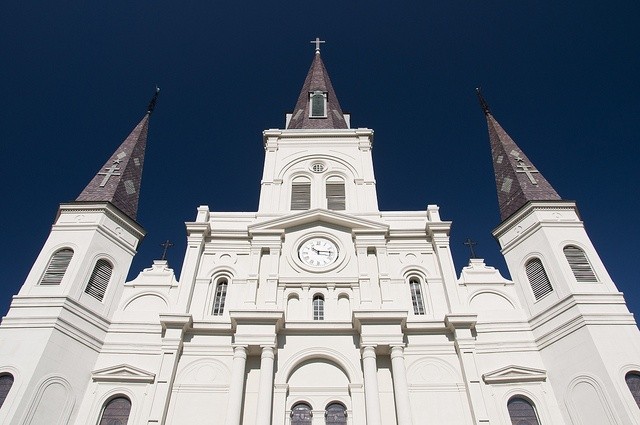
[299,237,339,267]
